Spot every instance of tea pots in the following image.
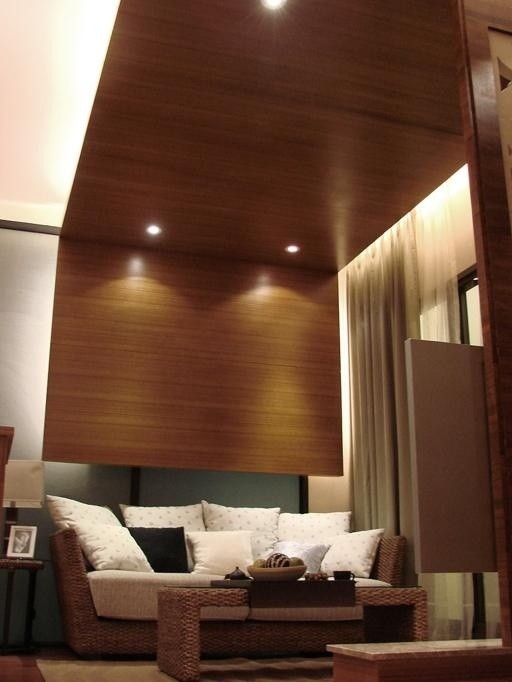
[223,567,250,580]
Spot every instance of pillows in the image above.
[272,542,332,576]
[187,531,250,574]
[279,511,351,539]
[201,500,281,561]
[320,528,386,577]
[118,503,207,573]
[128,527,189,572]
[67,520,153,571]
[47,493,122,528]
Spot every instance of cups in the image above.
[333,571,355,580]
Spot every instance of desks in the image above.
[1,560,44,656]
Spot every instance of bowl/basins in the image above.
[247,565,307,582]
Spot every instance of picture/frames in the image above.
[7,523,38,558]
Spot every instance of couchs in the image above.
[43,493,410,655]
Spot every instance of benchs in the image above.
[324,633,511,681]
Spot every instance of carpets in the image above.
[35,654,182,682]
